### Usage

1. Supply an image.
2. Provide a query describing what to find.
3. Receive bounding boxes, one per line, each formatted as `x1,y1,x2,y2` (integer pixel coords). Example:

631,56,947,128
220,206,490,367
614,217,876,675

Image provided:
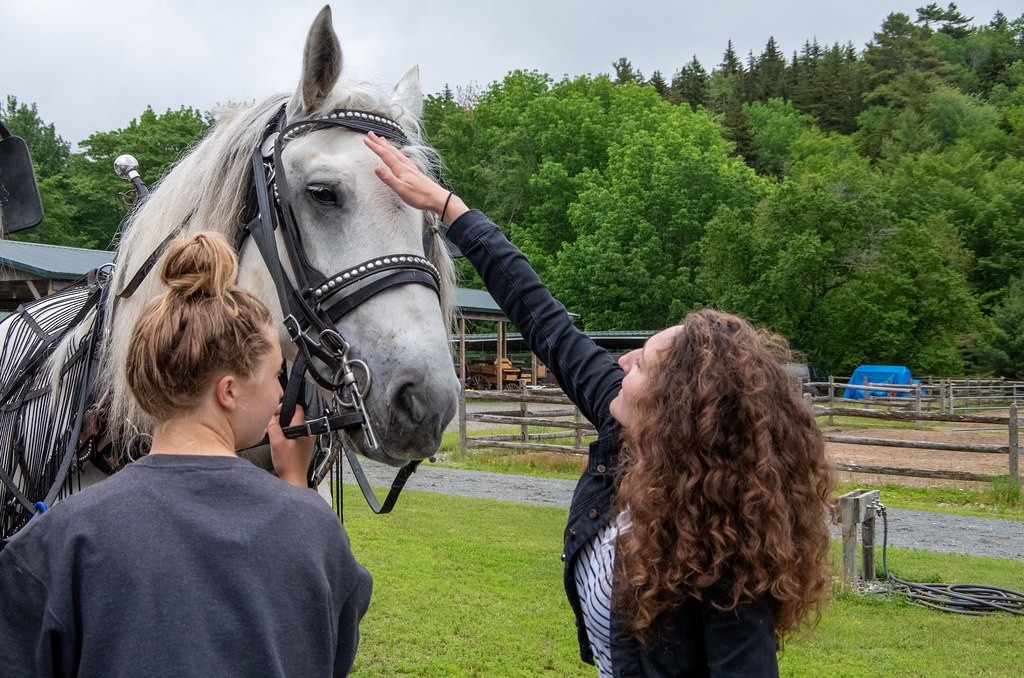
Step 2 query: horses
0,3,463,546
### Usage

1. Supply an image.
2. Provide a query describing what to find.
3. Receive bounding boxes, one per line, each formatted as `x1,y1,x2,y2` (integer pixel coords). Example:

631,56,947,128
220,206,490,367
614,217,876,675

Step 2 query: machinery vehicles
465,357,523,391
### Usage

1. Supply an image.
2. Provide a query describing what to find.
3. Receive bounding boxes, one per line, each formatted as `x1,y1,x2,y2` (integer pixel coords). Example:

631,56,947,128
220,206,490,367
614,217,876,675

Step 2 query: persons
0,230,376,678
360,125,845,678
